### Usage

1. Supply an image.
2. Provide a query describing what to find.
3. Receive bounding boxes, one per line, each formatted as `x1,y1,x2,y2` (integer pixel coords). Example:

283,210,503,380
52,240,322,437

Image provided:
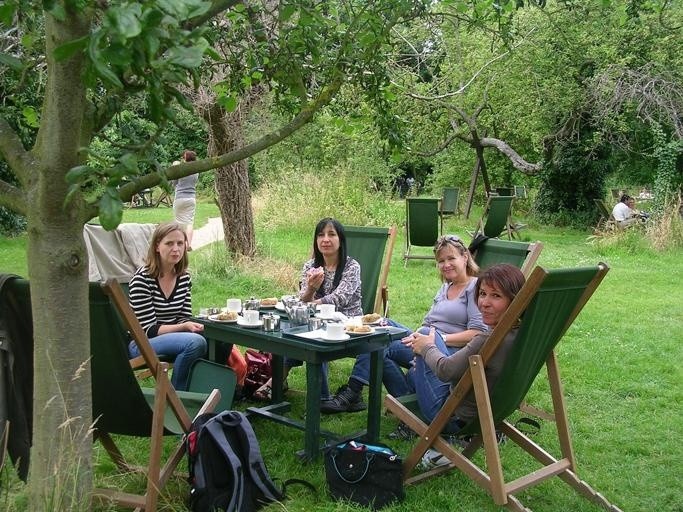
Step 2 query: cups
241,295,335,335
226,299,241,311
326,322,345,338
206,308,220,314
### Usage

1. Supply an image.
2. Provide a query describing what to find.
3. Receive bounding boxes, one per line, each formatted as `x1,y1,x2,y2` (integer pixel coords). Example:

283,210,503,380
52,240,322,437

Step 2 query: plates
208,314,240,323
352,314,383,325
343,325,375,335
219,308,241,313
319,333,350,342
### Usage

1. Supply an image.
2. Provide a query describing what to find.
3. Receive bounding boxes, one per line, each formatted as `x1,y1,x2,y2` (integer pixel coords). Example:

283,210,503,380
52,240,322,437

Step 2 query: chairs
288,224,398,398
0,273,222,511
463,184,530,241
404,196,444,268
438,187,461,220
84,222,159,382
383,261,626,512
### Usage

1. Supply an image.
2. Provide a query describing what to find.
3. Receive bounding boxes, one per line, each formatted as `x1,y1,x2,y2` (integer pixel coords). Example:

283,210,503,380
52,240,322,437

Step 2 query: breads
364,312,382,323
216,310,238,321
354,326,370,333
261,297,278,305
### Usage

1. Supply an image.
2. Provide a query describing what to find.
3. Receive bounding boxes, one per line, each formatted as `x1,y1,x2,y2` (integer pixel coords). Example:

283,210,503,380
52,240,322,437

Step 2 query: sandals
253,378,289,401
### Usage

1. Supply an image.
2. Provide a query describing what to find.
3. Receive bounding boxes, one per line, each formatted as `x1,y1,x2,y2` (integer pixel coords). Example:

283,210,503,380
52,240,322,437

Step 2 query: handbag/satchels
323,438,405,512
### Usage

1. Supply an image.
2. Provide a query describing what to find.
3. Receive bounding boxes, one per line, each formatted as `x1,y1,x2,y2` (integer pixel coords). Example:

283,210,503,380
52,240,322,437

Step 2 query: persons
252,217,363,415
628,196,636,209
410,264,529,468
128,222,209,393
323,236,486,418
406,176,416,192
172,150,199,251
610,194,637,228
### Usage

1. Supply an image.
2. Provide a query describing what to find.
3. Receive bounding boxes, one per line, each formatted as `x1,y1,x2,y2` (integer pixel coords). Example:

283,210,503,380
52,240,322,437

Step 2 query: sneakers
384,421,418,443
412,448,453,473
319,384,366,415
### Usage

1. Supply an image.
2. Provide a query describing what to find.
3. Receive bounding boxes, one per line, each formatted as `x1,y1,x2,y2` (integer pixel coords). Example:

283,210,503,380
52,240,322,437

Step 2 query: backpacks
185,410,286,511
